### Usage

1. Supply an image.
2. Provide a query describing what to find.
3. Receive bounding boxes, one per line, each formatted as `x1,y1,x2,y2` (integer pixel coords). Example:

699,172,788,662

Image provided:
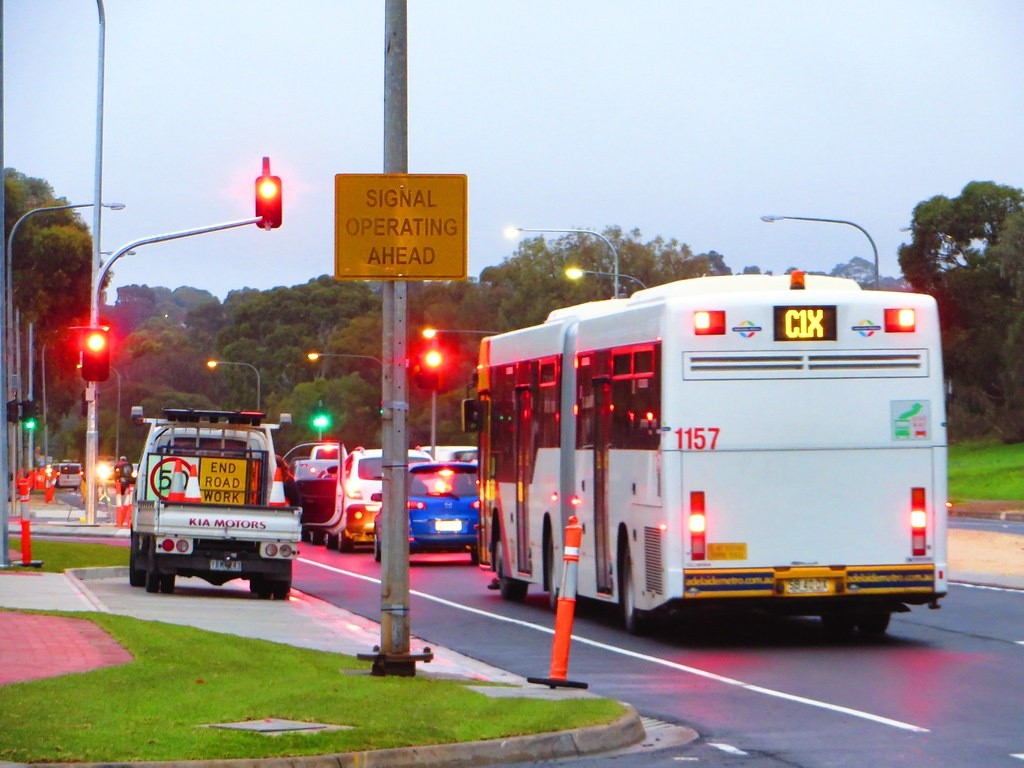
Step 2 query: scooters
112,466,134,494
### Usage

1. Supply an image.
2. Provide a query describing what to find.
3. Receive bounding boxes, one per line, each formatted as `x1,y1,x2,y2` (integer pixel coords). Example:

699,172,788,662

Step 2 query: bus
462,268,951,636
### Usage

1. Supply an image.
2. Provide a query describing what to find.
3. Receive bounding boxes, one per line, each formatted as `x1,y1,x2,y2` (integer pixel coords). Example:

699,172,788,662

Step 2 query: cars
278,443,479,566
36,453,139,494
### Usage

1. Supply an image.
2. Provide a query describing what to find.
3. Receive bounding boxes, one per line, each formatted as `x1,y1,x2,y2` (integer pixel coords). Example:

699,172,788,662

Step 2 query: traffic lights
23,418,38,432
79,326,111,384
255,176,282,230
310,411,333,433
422,348,444,372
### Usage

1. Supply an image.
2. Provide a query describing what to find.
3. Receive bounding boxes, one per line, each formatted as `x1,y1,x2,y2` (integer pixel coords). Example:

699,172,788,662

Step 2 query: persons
114,455,133,494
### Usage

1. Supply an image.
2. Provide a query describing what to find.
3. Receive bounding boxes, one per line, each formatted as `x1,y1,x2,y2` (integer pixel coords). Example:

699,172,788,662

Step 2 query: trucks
127,406,306,602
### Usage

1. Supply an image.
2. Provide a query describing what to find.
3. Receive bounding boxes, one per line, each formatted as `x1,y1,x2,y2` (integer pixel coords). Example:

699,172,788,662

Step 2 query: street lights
514,223,625,299
206,353,264,414
38,323,117,466
6,199,126,480
760,210,883,289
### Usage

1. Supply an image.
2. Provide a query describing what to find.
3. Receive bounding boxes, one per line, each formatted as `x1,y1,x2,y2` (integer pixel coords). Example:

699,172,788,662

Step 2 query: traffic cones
168,459,202,506
269,466,287,507
